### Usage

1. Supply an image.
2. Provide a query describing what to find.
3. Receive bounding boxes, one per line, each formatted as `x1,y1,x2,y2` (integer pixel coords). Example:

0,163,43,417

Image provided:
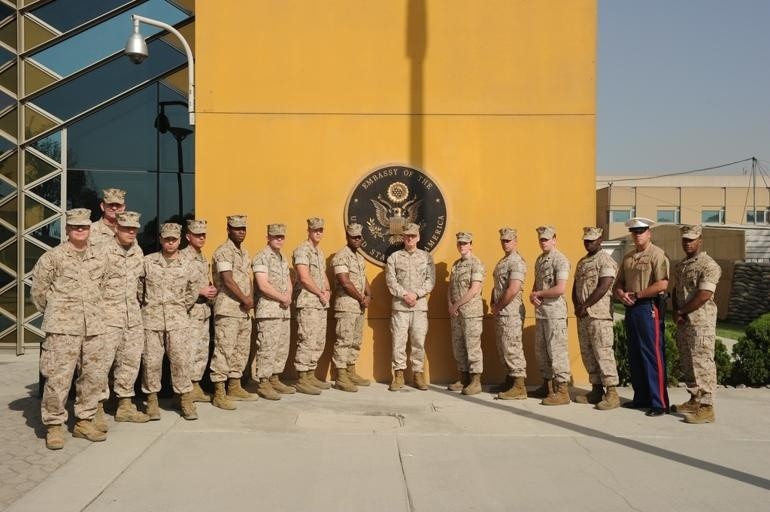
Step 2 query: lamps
125,13,195,126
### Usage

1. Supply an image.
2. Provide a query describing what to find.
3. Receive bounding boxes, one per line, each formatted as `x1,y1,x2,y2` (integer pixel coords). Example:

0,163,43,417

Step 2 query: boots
448,374,623,409
46,388,160,449
413,371,427,389
389,370,405,390
178,365,370,419
670,395,715,424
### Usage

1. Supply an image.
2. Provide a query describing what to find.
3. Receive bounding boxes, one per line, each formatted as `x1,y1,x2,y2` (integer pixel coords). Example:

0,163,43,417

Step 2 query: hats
456,232,472,243
159,224,182,239
269,224,286,236
307,218,324,230
65,207,94,226
402,224,420,235
499,228,516,241
187,220,207,235
536,226,555,240
227,216,247,228
347,224,363,237
103,189,125,204
582,227,603,241
680,225,703,239
626,218,654,232
115,212,141,228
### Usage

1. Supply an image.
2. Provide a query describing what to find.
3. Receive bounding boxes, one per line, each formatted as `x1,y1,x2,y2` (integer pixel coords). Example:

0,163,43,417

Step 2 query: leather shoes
645,407,667,416
622,400,644,409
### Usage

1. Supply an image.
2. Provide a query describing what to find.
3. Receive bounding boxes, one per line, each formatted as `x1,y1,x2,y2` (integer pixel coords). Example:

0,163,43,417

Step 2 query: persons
572,225,622,410
88,211,150,434
251,223,295,400
447,231,485,395
528,224,572,405
330,223,372,394
90,188,138,247
138,221,201,421
671,224,723,425
614,216,672,417
292,217,333,395
210,214,259,410
178,219,217,401
384,222,437,392
490,226,529,400
31,207,107,449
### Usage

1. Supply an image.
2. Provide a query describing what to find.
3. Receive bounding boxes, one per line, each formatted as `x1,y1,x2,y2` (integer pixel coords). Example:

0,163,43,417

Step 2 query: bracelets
632,292,640,302
678,306,681,314
